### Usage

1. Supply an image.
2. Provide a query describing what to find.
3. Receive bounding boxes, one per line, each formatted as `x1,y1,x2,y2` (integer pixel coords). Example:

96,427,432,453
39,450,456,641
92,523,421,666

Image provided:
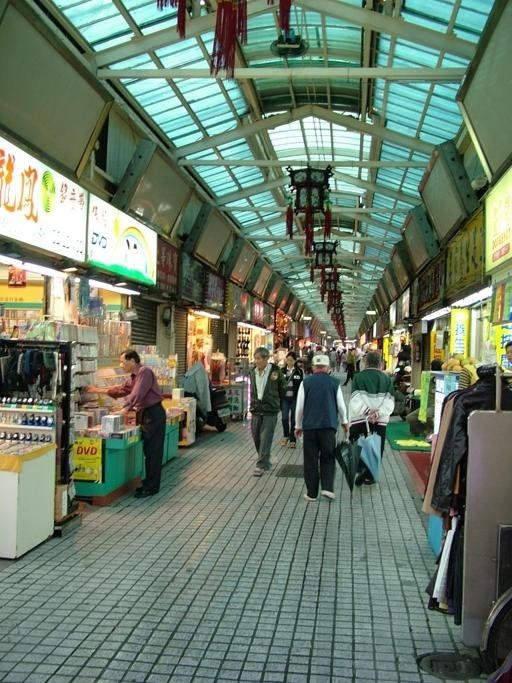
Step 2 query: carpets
383,422,432,500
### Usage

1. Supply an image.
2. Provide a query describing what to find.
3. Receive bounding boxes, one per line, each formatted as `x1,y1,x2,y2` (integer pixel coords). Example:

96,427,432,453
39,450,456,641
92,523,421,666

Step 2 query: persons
86,348,167,497
244,345,395,502
504,340,512,367
393,359,405,375
403,359,411,375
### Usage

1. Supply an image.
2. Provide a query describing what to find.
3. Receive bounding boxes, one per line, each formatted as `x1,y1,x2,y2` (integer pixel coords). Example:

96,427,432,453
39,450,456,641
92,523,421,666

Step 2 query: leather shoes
135,486,160,499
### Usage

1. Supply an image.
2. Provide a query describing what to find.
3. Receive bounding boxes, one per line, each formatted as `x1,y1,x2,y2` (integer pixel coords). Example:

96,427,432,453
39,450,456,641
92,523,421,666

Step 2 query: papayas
441,354,478,372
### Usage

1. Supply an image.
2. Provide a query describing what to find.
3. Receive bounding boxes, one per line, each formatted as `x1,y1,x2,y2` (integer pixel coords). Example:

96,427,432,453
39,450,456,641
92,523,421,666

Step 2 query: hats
311,354,331,368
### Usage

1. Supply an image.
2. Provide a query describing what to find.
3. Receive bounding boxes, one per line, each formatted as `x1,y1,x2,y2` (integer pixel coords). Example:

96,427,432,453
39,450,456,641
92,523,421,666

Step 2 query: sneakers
303,494,317,501
320,489,336,500
280,436,296,449
254,466,269,477
354,468,375,485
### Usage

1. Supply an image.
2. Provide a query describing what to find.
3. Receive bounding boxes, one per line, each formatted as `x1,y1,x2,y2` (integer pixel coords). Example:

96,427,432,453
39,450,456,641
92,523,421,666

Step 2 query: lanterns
327,293,349,347
286,164,333,221
310,240,337,269
319,272,340,292
